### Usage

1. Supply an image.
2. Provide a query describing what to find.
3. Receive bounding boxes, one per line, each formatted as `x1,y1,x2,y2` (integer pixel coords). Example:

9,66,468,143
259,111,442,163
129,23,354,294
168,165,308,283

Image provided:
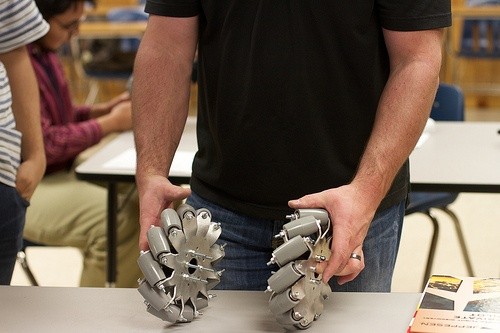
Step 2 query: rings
350,253,361,260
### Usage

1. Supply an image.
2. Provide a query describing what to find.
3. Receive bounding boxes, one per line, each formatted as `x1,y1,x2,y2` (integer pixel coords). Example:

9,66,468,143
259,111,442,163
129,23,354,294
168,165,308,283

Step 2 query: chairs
402,83,476,292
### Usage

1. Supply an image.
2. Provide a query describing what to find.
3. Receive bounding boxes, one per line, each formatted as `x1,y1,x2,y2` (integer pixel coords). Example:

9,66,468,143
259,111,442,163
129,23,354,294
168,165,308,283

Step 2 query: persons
129,1,453,289
22,5,141,288
1,1,50,287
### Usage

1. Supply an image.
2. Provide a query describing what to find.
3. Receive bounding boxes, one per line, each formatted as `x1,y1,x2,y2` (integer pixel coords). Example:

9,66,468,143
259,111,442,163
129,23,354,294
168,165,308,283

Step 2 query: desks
75,116,500,294
0,285,423,333
